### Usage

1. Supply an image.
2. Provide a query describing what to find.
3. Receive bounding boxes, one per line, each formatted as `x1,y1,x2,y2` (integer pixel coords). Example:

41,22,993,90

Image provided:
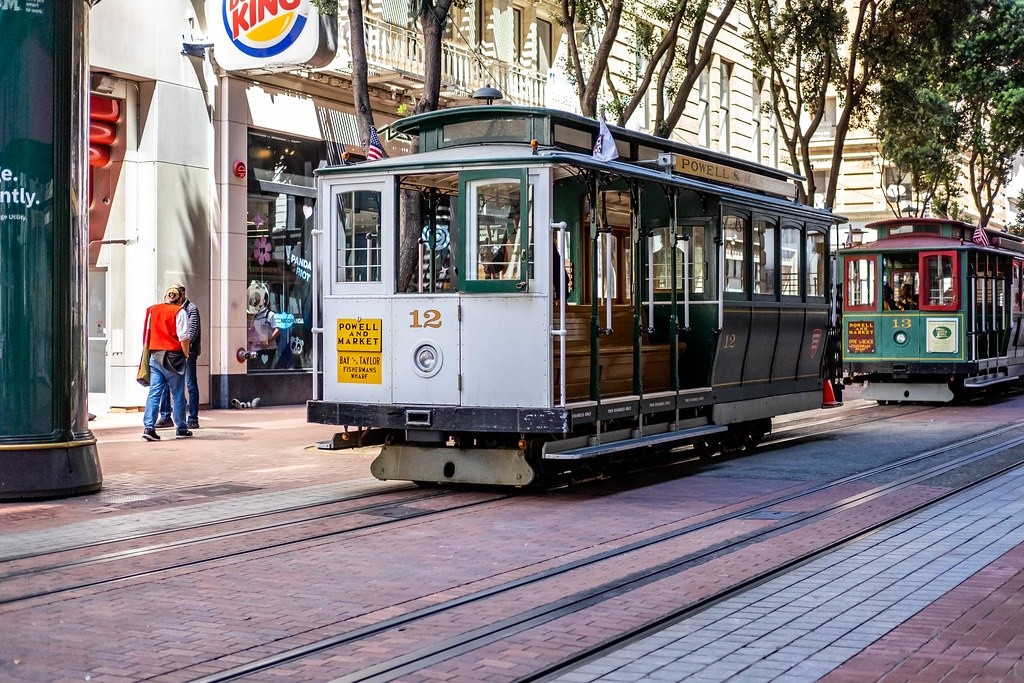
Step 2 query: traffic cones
823,378,838,407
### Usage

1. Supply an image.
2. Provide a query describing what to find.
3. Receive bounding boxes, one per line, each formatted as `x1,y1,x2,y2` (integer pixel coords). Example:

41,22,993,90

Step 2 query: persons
883,283,899,310
248,289,280,368
898,284,917,310
142,287,193,442
153,283,201,429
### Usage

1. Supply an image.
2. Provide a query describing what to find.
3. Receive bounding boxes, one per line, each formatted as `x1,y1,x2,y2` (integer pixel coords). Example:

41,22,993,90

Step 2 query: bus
839,217,1024,405
250,230,313,368
304,85,847,495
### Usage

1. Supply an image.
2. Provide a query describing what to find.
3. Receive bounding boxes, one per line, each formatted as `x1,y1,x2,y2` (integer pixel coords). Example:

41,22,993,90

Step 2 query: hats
163,287,181,303
245,283,270,314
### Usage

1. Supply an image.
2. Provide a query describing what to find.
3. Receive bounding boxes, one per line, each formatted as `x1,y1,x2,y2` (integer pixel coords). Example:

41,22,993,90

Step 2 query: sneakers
186,421,199,429
175,429,194,438
153,418,174,428
141,428,161,441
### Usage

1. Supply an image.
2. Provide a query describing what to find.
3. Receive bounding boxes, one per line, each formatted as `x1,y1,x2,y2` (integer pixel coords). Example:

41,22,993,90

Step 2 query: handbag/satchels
136,345,151,387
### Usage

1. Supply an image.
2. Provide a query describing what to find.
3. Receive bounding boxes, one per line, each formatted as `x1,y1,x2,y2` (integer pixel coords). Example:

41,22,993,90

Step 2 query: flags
591,114,619,162
367,127,383,161
973,220,989,247
846,226,853,245
